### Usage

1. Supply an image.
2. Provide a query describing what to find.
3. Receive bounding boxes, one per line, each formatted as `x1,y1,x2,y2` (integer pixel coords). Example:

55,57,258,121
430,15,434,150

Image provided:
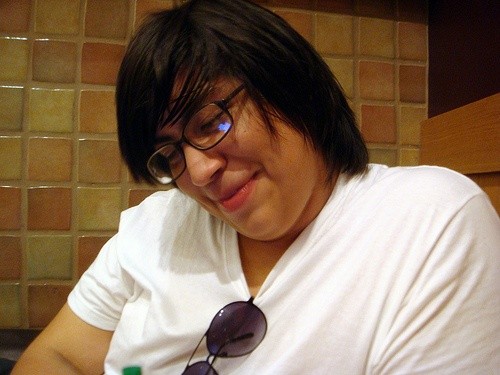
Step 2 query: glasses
147,82,249,187
181,296,267,375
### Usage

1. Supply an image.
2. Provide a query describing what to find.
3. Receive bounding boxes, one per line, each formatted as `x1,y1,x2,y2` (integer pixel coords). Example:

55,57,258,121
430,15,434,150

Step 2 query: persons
7,1,500,375
